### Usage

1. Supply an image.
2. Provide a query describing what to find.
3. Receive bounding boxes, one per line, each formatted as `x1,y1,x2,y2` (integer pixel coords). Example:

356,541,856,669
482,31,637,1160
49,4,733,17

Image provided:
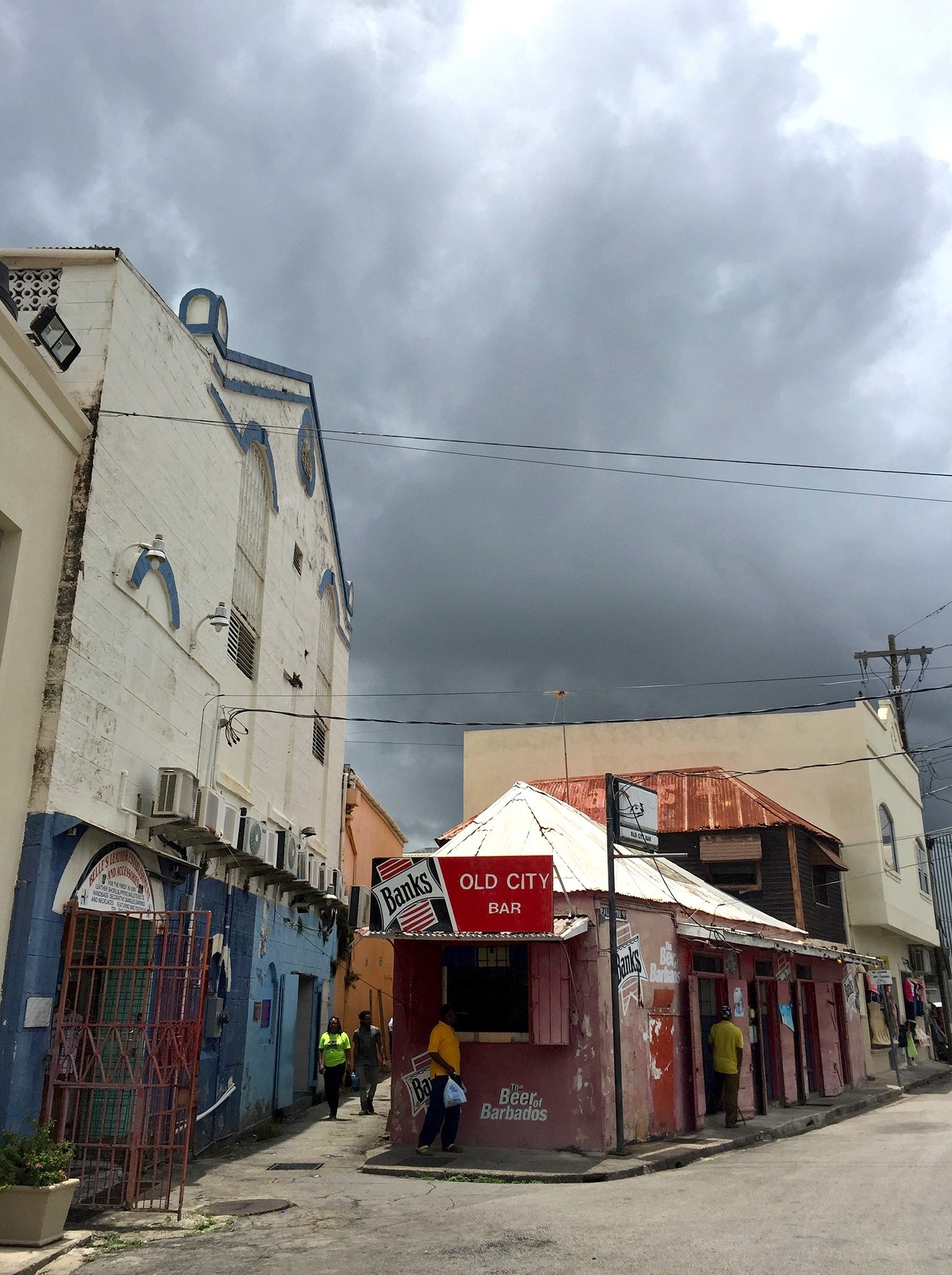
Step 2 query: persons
318,1016,352,1121
905,1021,919,1068
353,1010,387,1115
708,1005,744,1130
416,1005,464,1156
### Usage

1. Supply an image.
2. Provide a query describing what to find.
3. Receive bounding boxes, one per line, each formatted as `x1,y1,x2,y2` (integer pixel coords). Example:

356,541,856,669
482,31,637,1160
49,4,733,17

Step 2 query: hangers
907,1027,911,1033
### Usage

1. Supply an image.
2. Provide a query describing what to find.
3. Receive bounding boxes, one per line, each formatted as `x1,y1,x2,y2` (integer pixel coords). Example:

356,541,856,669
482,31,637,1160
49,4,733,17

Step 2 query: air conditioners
153,767,346,901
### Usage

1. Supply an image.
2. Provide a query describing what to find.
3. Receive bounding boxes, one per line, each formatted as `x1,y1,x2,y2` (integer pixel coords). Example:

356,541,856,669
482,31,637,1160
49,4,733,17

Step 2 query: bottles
352,1072,358,1087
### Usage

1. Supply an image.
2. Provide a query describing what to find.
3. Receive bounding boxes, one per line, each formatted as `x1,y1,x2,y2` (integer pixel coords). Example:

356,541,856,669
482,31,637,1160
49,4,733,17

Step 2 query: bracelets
383,1057,385,1060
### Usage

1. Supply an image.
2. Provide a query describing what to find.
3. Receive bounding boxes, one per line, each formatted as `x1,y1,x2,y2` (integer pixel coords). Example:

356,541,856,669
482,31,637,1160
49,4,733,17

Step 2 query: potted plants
0,1115,80,1248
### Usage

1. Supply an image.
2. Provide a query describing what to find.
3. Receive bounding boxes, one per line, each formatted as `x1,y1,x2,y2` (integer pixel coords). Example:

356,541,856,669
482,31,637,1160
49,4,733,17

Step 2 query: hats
720,1006,732,1018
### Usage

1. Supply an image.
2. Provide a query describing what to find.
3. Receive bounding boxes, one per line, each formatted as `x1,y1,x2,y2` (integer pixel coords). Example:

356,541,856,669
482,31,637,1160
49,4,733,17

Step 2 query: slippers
415,1145,433,1155
442,1144,462,1153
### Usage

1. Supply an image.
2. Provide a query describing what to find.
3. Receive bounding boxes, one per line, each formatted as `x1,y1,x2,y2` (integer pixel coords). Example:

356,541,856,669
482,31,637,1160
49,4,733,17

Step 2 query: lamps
303,882,337,905
114,533,166,576
191,601,228,649
22,306,82,374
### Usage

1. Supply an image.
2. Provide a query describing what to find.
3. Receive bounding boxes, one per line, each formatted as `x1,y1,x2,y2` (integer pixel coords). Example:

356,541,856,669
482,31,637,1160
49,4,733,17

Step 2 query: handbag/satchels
351,1072,358,1088
443,1076,468,1108
344,1074,353,1088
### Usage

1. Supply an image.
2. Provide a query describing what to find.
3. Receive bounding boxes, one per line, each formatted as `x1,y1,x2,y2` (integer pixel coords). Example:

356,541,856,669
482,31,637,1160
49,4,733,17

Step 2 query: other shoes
367,1102,374,1113
326,1113,336,1121
725,1122,739,1128
359,1107,368,1115
